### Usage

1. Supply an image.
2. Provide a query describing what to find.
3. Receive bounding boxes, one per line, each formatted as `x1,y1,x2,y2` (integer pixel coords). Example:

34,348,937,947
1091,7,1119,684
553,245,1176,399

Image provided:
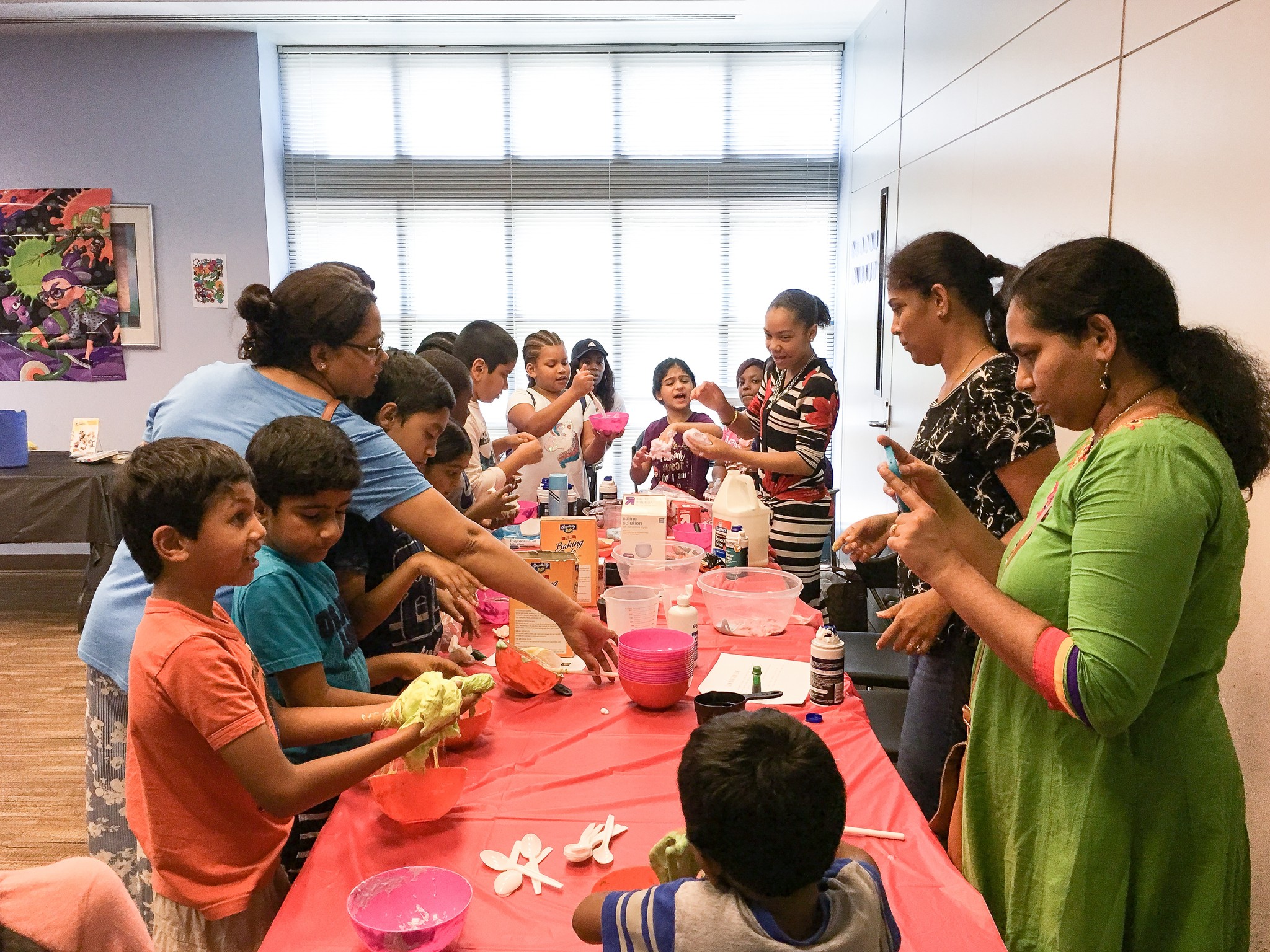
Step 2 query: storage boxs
509,550,579,658
668,485,703,528
540,516,598,607
620,494,668,562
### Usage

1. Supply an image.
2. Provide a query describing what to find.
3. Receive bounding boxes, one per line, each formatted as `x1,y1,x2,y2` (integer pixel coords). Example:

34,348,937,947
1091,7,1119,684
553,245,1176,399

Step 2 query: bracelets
719,406,738,426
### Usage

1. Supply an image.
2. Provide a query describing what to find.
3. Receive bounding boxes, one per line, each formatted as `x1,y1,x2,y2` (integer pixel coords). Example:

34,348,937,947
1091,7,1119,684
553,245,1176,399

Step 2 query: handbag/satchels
928,737,967,873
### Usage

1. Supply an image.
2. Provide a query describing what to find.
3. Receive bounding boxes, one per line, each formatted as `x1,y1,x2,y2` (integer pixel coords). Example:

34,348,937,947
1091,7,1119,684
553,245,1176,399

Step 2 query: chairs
837,627,912,760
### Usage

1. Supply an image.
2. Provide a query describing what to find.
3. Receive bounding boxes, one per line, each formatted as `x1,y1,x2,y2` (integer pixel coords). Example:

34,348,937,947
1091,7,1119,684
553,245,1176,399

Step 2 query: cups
603,505,622,537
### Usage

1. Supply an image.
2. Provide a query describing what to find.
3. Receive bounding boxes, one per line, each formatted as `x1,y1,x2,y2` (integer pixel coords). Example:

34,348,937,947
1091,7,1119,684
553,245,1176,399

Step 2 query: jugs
600,585,670,638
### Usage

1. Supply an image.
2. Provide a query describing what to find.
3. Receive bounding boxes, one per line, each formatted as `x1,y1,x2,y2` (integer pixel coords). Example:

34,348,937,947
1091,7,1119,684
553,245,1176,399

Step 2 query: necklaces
767,349,817,410
1087,384,1167,451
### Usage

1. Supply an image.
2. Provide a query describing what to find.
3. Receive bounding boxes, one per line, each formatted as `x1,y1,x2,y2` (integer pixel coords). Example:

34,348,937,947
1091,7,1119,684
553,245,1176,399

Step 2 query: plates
591,866,660,894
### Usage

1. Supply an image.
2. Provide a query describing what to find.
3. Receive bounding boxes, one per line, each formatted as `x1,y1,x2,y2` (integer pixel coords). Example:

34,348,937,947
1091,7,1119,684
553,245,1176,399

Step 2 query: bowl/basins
346,866,473,952
589,412,629,436
671,523,712,549
704,489,760,502
697,567,804,638
611,540,706,601
496,638,565,696
513,501,538,524
474,588,510,625
368,767,467,824
618,628,695,709
439,695,493,751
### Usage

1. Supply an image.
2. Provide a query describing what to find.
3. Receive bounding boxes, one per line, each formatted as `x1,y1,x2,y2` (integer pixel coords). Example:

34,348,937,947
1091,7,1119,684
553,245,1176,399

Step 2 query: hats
571,338,608,361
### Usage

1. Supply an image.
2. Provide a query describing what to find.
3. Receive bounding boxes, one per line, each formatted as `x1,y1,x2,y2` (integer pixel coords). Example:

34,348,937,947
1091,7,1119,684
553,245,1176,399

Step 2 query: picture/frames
111,204,161,349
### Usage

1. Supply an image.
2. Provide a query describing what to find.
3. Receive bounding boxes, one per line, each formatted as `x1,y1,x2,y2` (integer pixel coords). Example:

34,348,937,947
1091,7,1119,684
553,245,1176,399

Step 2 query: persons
686,289,840,629
414,319,544,554
231,416,468,882
572,707,901,952
118,439,482,951
77,266,619,938
324,347,481,695
878,237,1270,952
565,339,627,468
505,330,624,502
704,358,764,503
832,232,1061,850
630,358,723,501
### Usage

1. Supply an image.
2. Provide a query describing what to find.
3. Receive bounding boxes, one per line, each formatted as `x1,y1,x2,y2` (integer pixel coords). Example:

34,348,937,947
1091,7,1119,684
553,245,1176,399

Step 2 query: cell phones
885,445,909,513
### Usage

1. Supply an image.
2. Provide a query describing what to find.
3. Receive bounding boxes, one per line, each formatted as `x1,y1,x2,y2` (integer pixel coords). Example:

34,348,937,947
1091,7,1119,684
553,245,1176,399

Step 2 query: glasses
333,331,386,356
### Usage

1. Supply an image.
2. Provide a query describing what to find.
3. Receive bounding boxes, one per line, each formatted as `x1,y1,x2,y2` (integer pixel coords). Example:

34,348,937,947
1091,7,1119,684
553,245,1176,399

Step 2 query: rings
891,523,899,534
910,641,920,649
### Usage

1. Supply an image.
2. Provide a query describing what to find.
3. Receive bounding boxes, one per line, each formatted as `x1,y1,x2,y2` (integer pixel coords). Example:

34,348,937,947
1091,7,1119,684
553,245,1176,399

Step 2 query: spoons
520,833,542,894
479,849,563,888
494,847,553,894
563,814,628,864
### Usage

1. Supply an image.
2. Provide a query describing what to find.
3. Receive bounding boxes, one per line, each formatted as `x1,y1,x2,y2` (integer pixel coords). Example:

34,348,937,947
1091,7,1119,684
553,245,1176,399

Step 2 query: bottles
668,584,698,668
599,476,617,501
725,525,749,581
752,666,761,693
711,467,770,567
809,625,844,707
536,473,577,518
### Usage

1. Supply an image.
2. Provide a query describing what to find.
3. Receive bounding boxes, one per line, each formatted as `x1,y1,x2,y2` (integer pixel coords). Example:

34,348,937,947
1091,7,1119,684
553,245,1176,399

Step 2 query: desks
258,560,1005,952
0,451,122,634
